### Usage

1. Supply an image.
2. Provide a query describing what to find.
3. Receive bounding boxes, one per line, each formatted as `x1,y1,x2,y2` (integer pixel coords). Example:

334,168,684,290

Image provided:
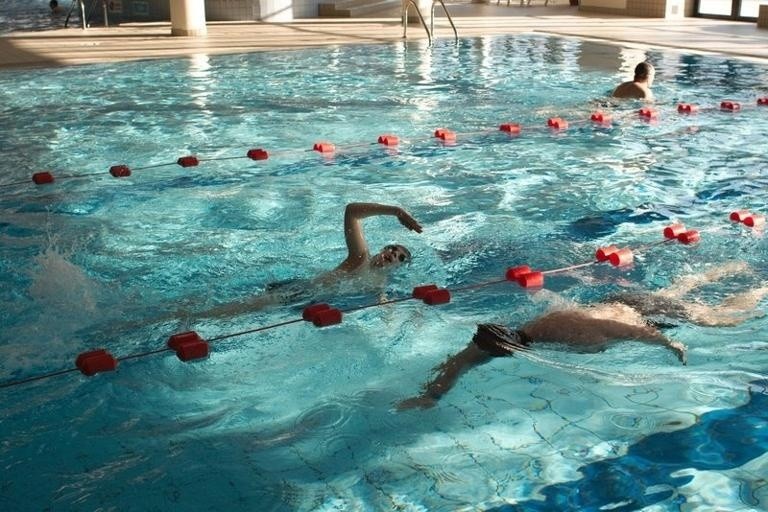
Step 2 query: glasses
385,245,408,263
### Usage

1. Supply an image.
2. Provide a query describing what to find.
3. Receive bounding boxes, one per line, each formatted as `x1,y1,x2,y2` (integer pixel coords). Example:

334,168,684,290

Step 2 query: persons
395,260,767,411
613,62,655,101
105,202,422,335
